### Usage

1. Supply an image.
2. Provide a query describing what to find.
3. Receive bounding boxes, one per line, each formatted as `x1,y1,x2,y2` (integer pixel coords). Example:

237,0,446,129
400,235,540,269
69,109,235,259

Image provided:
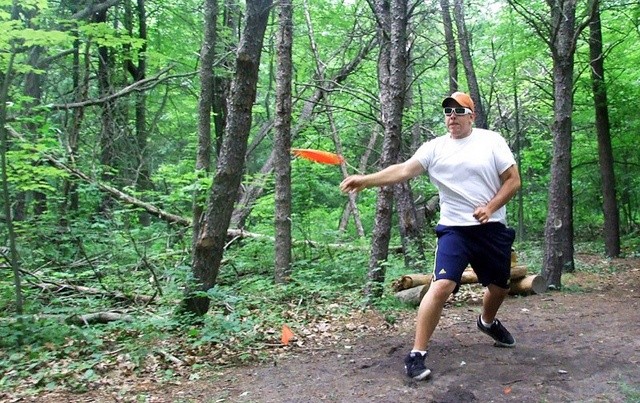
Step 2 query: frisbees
289,147,343,165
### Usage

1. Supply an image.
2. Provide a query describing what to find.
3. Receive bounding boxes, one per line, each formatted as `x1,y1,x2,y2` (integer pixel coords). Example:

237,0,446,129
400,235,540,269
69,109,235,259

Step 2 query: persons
339,91,522,382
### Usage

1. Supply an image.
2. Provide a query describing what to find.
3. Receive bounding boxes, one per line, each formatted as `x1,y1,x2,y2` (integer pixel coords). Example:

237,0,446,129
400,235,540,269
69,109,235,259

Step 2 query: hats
442,92,474,112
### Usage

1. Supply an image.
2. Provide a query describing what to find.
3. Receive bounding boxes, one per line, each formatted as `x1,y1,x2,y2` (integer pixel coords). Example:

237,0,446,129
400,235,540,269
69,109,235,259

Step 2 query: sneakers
404,350,431,381
476,314,516,347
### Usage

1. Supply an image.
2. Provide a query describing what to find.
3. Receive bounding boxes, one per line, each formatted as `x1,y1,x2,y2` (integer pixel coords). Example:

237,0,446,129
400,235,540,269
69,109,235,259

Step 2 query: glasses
442,106,472,116
